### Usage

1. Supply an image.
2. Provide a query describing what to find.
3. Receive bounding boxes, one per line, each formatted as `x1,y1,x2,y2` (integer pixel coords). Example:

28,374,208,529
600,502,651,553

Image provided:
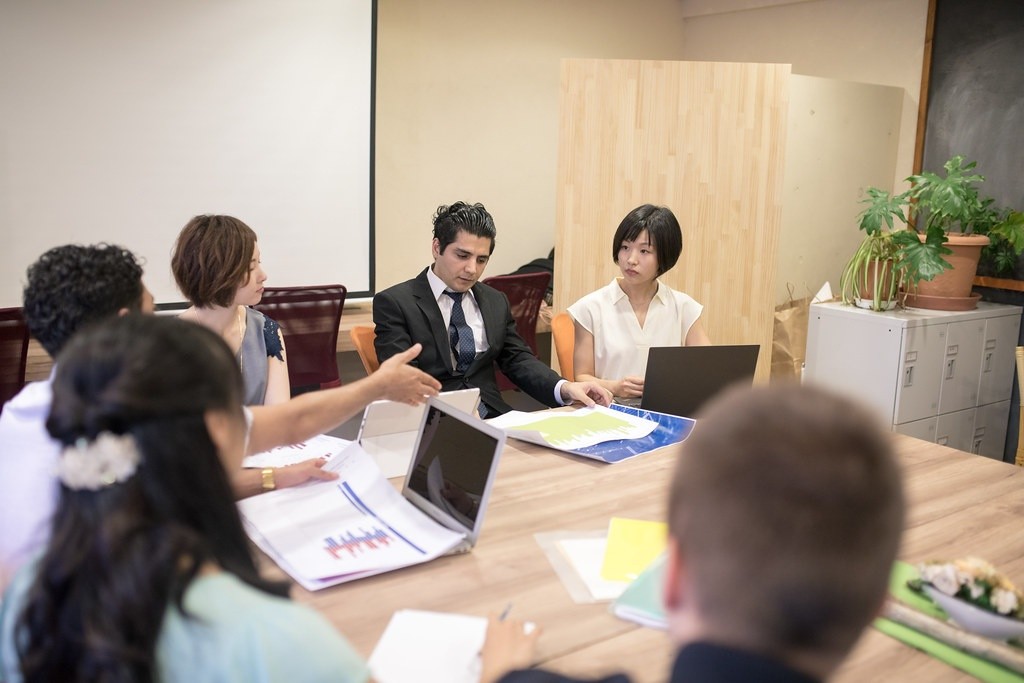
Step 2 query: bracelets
259,467,275,492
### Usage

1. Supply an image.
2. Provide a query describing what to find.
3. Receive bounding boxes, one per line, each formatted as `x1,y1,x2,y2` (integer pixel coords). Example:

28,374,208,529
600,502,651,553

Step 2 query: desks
280,297,551,375
249,406,1024,683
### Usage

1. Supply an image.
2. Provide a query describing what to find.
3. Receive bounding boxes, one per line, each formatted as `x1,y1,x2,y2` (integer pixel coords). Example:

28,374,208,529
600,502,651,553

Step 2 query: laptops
401,395,506,557
611,345,761,421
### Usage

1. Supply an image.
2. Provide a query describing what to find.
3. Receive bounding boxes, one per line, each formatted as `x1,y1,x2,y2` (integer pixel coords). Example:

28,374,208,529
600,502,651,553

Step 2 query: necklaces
193,303,243,375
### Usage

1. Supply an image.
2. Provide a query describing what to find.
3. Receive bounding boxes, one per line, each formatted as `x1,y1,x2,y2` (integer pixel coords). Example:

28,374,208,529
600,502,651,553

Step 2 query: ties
442,290,488,421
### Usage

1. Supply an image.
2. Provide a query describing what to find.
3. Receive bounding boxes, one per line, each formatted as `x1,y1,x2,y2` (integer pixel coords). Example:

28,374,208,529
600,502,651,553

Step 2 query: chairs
247,283,345,396
482,271,552,391
0,307,31,416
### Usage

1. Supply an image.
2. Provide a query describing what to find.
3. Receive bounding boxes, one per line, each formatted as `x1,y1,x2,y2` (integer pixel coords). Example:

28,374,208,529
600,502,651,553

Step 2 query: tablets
358,388,480,446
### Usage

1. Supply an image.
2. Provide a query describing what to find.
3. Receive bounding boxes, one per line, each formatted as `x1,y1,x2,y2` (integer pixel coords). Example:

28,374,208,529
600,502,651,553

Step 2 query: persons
163,214,292,407
373,201,614,420
0,240,442,561
1,311,381,683
488,369,906,683
567,203,709,400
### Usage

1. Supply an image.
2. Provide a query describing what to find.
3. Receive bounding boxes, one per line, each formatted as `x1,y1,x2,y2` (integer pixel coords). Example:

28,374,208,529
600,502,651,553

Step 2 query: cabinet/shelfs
806,296,1023,462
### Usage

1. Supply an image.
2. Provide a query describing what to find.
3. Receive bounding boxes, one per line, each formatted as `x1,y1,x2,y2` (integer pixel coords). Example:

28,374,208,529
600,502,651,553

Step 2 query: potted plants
852,150,1013,311
840,231,902,309
